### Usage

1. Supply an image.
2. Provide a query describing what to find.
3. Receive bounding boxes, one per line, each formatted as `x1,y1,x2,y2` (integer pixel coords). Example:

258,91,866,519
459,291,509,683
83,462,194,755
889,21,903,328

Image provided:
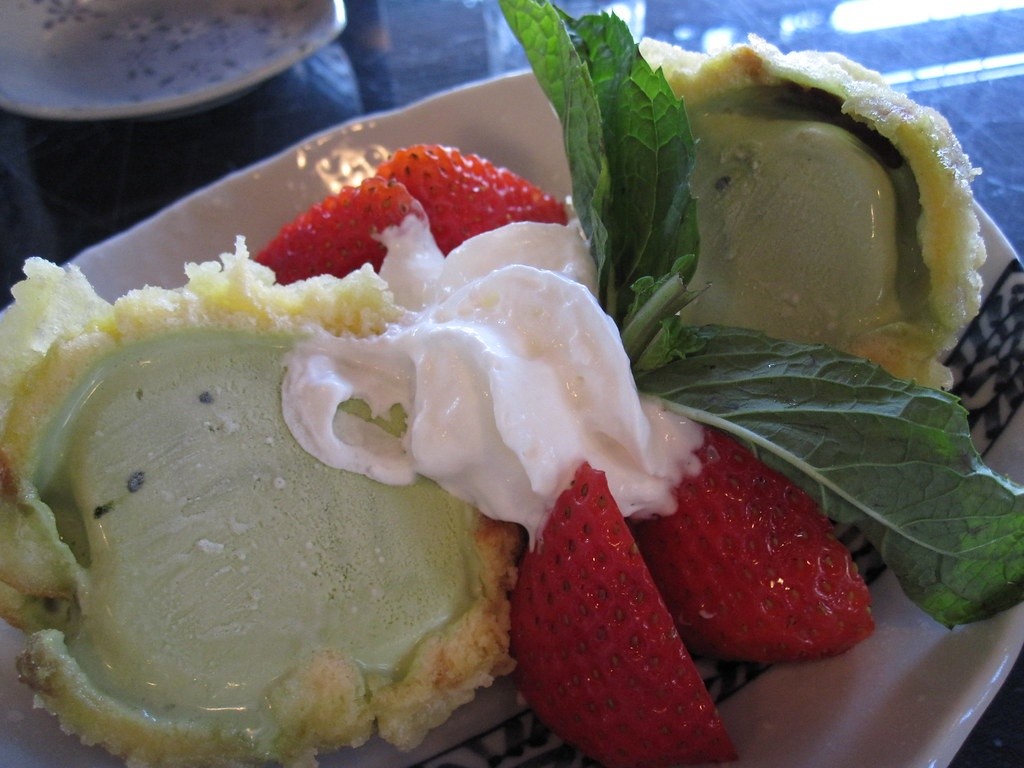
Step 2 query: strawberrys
248,175,431,286
506,459,735,768
637,398,877,670
368,145,573,256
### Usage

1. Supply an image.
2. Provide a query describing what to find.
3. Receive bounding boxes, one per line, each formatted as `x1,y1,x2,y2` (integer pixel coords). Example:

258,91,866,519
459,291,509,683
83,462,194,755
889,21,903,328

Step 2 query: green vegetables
506,0,1023,646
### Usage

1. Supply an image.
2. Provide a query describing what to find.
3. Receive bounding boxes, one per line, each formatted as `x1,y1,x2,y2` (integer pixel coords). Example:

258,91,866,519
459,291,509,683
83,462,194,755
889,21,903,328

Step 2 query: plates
1,0,349,125
0,67,1024,767
